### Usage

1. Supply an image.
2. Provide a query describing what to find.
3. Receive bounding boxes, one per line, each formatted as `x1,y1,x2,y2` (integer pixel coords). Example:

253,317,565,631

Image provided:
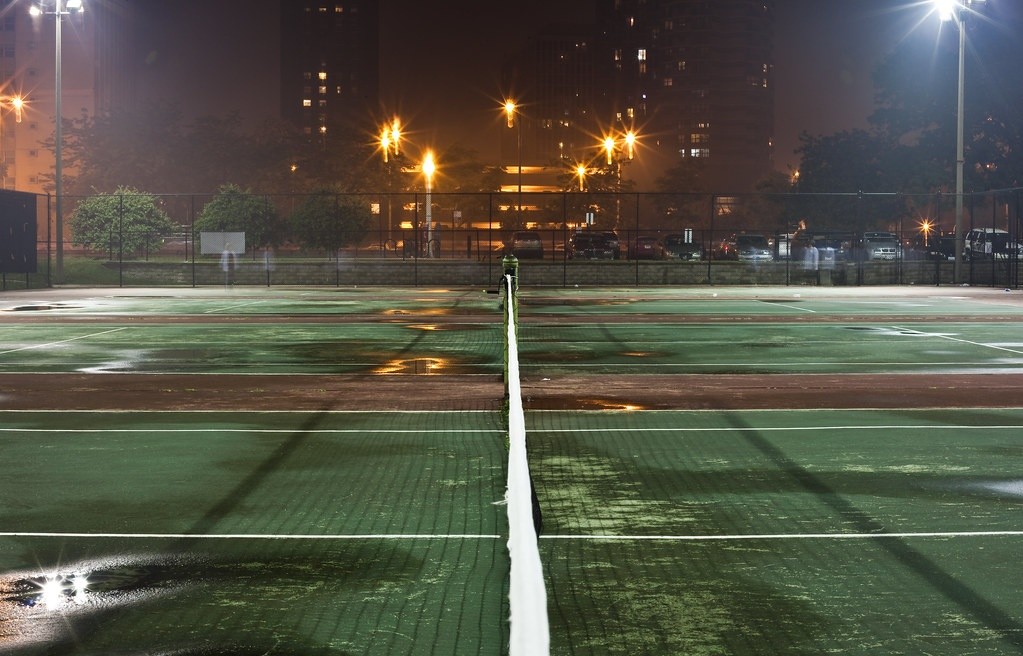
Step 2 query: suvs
507,230,548,261
902,227,1023,261
564,232,622,262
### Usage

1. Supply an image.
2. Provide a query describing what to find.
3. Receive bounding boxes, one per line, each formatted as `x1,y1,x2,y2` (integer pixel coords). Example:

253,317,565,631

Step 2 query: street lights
28,0,85,284
934,0,969,285
381,126,401,251
422,149,436,259
503,99,523,229
605,131,634,230
923,222,929,247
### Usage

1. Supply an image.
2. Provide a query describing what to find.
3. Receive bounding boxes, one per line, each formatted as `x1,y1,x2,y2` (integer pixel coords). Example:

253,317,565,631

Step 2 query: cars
626,234,705,264
767,232,905,264
714,234,773,263
